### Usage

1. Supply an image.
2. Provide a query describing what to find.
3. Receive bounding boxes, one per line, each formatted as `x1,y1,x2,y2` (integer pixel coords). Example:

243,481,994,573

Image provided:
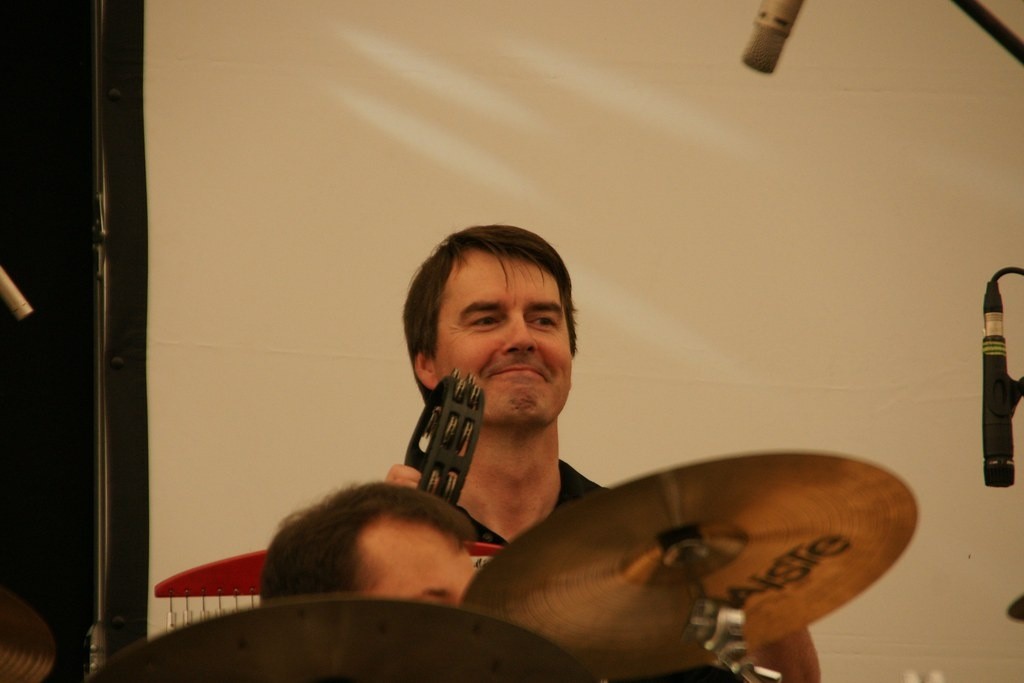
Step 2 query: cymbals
81,592,596,683
0,587,56,683
403,368,485,506
458,449,920,680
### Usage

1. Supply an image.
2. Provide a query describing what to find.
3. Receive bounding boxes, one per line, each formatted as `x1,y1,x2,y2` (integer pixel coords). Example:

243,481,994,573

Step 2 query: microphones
981,281,1016,491
743,1,804,74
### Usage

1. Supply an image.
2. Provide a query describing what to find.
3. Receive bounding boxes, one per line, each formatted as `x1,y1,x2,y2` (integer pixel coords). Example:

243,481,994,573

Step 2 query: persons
382,226,824,683
257,479,478,608
